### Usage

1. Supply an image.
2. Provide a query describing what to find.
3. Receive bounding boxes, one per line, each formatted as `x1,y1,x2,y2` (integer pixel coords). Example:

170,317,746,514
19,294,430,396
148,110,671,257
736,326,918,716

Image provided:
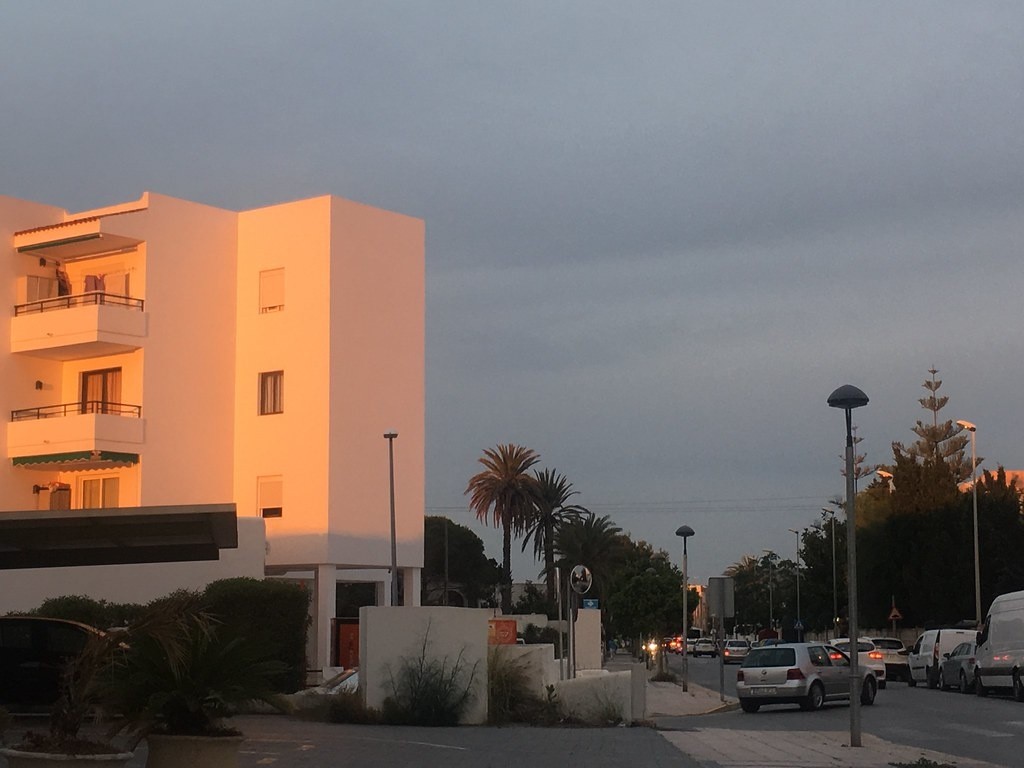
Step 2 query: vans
975,590,1024,700
906,629,977,687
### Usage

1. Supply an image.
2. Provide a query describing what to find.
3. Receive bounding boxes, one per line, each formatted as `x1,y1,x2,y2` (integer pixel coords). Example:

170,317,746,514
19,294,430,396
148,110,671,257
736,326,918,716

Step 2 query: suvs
826,638,888,690
736,642,878,714
862,636,909,681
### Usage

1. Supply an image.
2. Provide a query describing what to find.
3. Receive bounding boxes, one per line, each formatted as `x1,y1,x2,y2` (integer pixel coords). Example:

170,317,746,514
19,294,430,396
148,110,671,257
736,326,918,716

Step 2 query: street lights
382,429,400,605
675,525,696,693
823,507,839,639
825,384,869,749
788,528,803,644
954,418,982,632
763,549,773,631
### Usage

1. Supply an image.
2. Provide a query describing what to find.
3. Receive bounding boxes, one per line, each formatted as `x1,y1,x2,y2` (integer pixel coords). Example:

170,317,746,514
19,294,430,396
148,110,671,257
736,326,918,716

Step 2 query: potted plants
0,586,250,768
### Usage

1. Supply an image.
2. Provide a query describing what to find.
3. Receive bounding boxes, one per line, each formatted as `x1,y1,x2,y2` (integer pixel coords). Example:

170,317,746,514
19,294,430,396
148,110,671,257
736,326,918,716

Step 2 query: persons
614,636,619,655
609,637,615,660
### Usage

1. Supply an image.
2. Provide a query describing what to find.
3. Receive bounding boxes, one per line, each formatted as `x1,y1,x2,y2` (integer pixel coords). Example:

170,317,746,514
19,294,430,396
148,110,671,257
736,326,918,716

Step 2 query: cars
642,633,790,663
294,666,360,704
0,614,137,713
937,641,977,693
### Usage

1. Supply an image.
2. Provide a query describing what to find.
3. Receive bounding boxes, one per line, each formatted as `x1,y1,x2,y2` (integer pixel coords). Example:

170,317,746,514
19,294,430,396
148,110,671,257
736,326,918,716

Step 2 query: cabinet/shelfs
38,487,70,511
17,275,59,315
